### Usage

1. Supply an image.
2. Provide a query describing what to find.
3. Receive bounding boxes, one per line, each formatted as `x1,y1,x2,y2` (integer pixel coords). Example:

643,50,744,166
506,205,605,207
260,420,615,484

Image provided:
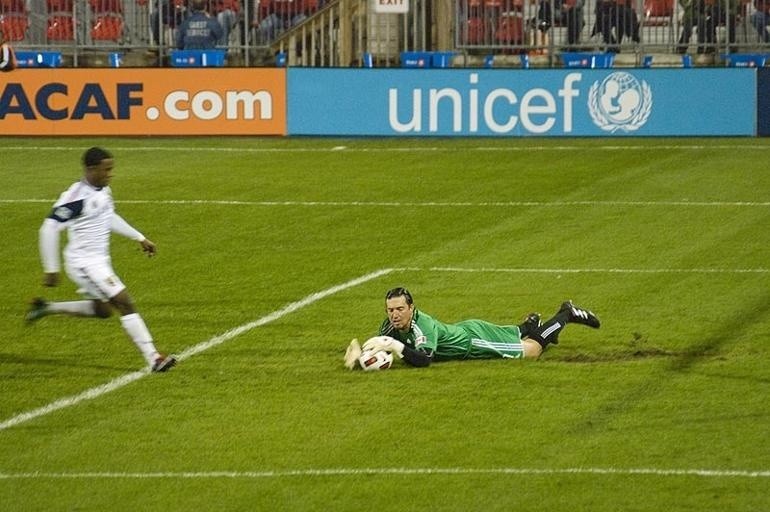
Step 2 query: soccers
359,343,393,371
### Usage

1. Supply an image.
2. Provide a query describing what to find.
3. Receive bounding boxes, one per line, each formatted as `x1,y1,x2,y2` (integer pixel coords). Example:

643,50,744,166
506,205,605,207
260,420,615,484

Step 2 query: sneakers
152,355,176,372
23,295,49,321
525,312,544,332
560,300,601,328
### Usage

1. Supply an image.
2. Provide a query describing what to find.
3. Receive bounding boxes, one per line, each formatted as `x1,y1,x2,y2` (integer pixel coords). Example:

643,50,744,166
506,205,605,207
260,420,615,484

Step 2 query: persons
1,30,20,73
24,148,177,371
463,1,641,53
343,287,601,372
152,1,322,50
643,1,768,54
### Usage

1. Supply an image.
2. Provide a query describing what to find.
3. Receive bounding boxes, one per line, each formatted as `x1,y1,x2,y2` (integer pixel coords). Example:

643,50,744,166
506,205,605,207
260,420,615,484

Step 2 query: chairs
460,0,677,52
0,1,322,43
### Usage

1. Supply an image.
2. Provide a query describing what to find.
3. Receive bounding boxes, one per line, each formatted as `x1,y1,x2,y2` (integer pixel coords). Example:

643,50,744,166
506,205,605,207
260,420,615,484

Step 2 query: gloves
361,335,405,359
343,338,362,370
